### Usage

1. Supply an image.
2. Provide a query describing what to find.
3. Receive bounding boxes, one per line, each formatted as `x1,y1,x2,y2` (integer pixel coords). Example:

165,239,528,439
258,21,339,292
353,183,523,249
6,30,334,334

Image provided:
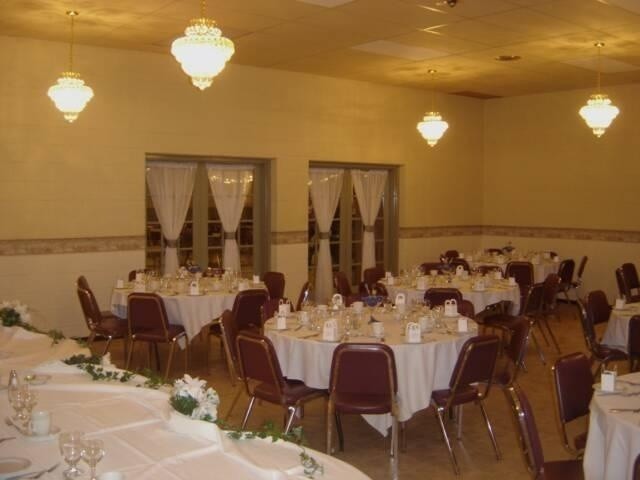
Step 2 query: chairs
218,246,638,476
77,266,286,382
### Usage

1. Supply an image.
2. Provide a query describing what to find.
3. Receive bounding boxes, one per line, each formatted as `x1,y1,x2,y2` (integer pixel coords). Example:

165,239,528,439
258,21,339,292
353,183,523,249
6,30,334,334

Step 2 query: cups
29,411,52,435
394,265,516,289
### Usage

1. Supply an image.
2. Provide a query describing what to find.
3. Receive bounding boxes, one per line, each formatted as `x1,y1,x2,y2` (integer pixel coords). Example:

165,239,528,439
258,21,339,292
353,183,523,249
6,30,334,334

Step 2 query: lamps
414,67,452,150
46,10,96,126
575,42,623,142
170,0,238,94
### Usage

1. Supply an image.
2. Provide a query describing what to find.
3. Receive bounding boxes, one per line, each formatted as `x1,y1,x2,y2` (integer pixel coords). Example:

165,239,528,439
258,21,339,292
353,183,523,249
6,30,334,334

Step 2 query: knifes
299,333,319,339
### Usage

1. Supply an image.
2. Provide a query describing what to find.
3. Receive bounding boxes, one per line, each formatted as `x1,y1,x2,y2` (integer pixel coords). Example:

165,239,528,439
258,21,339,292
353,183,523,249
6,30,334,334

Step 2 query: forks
615,380,640,386
6,418,27,435
598,391,633,398
5,462,60,480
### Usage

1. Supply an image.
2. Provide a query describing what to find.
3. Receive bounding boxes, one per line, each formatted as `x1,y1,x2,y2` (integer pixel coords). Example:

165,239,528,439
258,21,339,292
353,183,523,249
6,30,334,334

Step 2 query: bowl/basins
23,373,53,386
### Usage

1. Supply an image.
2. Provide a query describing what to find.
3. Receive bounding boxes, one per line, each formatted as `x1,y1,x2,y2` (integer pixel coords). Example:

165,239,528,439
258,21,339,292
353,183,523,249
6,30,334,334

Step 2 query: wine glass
79,439,107,480
143,265,241,295
303,294,445,339
57,431,85,480
7,383,38,430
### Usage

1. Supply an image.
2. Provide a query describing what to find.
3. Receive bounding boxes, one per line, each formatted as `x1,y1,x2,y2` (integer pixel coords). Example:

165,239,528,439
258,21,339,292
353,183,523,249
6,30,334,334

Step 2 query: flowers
1,297,30,325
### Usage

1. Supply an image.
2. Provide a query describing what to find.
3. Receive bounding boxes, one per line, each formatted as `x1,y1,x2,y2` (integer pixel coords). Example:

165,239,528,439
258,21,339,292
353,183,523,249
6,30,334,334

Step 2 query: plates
591,383,630,394
0,454,46,480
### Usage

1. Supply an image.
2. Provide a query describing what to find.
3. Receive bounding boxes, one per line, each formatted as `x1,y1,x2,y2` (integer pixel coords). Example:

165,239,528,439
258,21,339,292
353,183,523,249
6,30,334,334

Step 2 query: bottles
7,368,20,393
238,277,249,292
